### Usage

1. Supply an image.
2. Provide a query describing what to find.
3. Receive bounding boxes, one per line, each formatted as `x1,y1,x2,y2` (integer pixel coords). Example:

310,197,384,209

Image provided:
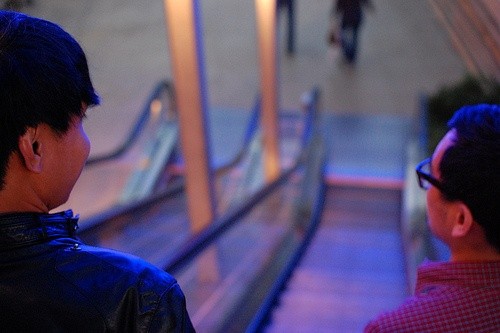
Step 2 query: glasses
417,159,448,196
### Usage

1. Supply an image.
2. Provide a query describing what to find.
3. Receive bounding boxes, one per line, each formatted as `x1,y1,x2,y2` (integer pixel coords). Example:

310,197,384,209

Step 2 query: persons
328,0,371,62
362,104,500,332
1,11,194,333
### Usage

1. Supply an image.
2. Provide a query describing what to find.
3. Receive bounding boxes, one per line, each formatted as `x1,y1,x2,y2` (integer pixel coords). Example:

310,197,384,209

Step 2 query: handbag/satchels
327,32,336,44
